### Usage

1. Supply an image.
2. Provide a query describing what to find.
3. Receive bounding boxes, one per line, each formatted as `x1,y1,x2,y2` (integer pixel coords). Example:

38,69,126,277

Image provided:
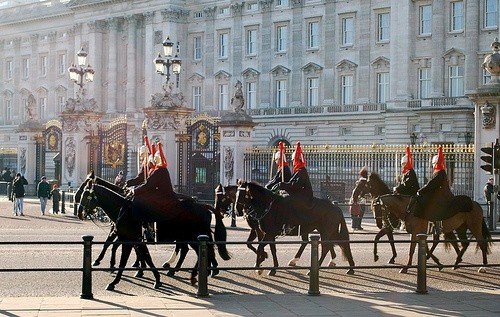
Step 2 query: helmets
432,147,444,173
148,144,159,170
154,142,168,169
275,143,289,168
291,141,306,173
140,136,152,162
401,147,413,173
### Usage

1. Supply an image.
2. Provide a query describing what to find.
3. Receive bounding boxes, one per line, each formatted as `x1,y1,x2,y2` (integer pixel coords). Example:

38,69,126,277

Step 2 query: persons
0,165,16,194
414,154,454,218
14,173,29,215
148,153,155,175
126,151,178,236
348,180,369,230
48,184,61,215
12,173,22,214
271,151,313,234
120,146,155,193
263,151,292,197
393,154,419,195
484,177,494,224
114,170,125,189
37,176,51,215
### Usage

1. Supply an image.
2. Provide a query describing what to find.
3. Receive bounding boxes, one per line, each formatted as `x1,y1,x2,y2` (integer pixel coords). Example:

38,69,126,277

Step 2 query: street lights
153,37,180,95
67,47,95,99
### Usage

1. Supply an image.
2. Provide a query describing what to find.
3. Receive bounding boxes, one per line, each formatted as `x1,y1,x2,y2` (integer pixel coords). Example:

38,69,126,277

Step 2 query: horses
74,170,233,292
213,179,355,275
349,165,497,273
